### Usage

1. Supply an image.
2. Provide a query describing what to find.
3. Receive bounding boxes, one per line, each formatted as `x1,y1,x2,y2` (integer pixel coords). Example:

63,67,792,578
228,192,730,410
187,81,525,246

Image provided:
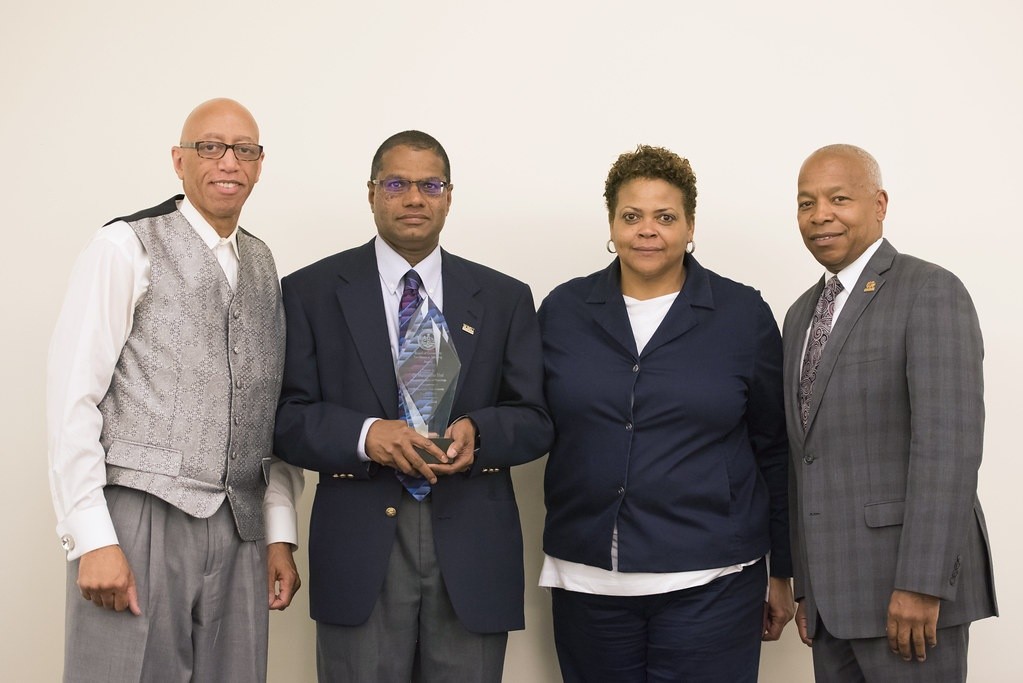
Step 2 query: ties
800,277,844,430
397,269,438,502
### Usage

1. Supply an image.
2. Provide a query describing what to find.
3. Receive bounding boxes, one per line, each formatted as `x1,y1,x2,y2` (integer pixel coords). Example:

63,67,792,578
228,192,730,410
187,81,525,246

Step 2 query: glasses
180,140,263,161
372,177,448,197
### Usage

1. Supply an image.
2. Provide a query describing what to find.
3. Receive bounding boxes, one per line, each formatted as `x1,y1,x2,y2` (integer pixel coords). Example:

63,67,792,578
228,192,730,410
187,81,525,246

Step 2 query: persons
782,143,999,683
274,129,554,683
538,146,796,683
49,98,305,683
